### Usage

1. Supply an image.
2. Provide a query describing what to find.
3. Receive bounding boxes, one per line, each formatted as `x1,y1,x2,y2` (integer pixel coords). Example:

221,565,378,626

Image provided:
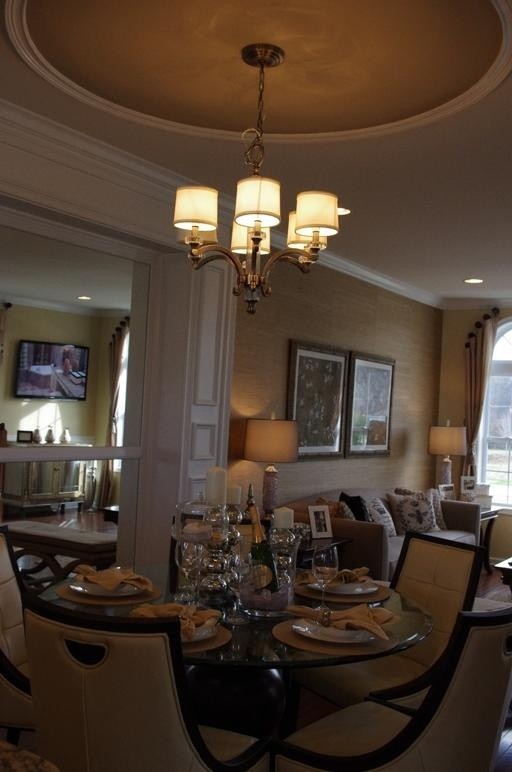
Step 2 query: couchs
279,487,480,581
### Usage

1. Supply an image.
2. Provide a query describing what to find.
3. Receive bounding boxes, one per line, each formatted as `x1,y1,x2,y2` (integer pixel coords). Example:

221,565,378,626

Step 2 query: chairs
0,525,37,743
289,528,484,706
268,608,511,772
22,606,273,772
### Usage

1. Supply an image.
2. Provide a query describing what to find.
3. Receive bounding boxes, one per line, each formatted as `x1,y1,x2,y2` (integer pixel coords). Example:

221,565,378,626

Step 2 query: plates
293,618,382,643
180,626,215,644
68,581,138,595
309,583,378,595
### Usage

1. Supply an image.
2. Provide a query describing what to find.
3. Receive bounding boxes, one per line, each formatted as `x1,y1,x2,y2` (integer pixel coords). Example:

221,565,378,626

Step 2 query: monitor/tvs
13,339,91,401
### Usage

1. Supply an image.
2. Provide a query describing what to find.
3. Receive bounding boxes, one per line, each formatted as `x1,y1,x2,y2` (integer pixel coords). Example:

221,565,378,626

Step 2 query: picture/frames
460,475,477,494
285,339,349,459
438,483,456,501
17,431,33,442
343,350,396,458
307,503,333,540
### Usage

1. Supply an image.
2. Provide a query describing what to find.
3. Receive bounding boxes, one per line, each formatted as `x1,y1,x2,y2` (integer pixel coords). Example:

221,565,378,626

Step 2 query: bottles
248,505,279,595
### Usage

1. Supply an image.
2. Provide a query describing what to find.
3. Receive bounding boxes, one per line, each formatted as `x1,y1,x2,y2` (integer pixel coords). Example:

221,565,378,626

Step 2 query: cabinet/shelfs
1,440,93,509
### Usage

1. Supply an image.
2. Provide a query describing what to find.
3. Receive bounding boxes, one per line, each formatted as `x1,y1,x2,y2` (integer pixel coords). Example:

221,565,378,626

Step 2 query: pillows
395,488,447,530
367,497,396,536
383,492,440,535
316,496,356,520
339,491,374,522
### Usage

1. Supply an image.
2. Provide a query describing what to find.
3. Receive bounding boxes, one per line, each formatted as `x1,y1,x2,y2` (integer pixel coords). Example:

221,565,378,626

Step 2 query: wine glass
312,546,338,613
175,542,204,602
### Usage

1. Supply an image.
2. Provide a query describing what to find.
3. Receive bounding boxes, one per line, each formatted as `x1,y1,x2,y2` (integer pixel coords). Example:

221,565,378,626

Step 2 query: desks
241,515,353,568
0,509,117,601
99,505,118,525
480,509,499,575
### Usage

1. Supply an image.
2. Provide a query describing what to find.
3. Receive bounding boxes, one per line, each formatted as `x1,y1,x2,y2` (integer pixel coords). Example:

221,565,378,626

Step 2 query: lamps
241,417,299,528
173,44,341,316
428,426,467,484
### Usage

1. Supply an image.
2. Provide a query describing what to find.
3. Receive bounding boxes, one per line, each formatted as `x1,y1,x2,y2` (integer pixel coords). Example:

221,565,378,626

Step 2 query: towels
71,563,154,594
128,602,223,640
296,566,372,587
283,601,401,643
